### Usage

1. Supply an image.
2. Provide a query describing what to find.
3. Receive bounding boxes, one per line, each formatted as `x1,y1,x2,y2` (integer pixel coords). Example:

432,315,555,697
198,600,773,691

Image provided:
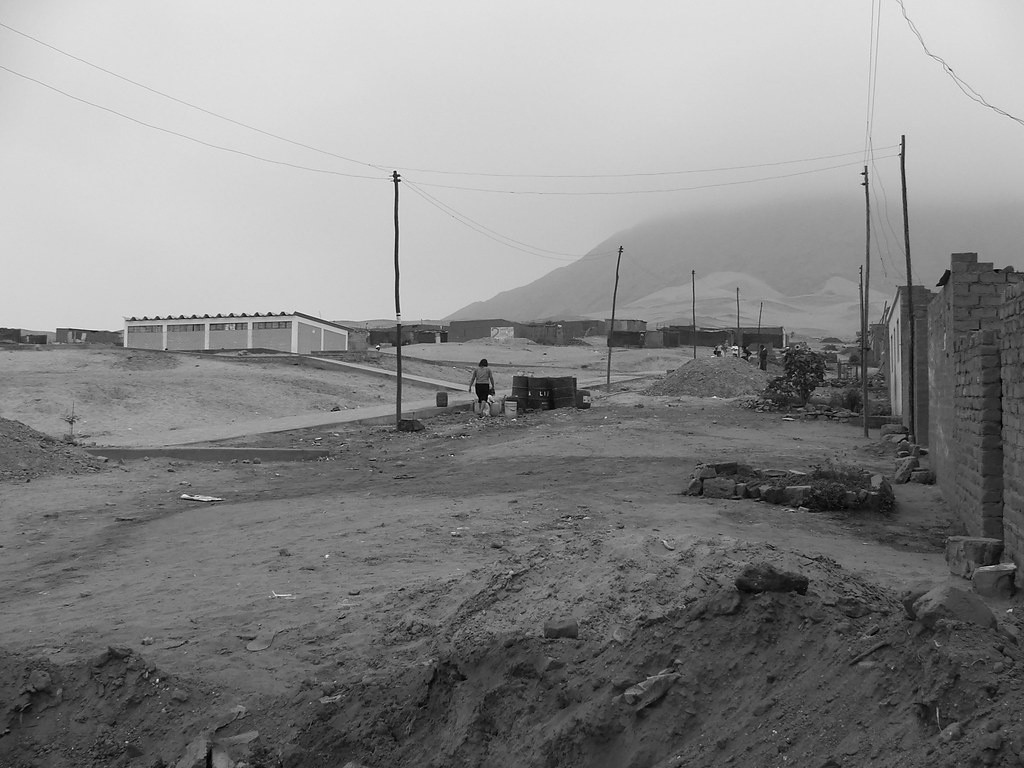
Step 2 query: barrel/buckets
505,402,517,419
512,375,591,408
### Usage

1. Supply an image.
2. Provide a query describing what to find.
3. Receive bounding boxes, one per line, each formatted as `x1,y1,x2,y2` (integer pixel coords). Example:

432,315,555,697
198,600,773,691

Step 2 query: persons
469,359,495,418
758,345,767,370
713,338,752,362
